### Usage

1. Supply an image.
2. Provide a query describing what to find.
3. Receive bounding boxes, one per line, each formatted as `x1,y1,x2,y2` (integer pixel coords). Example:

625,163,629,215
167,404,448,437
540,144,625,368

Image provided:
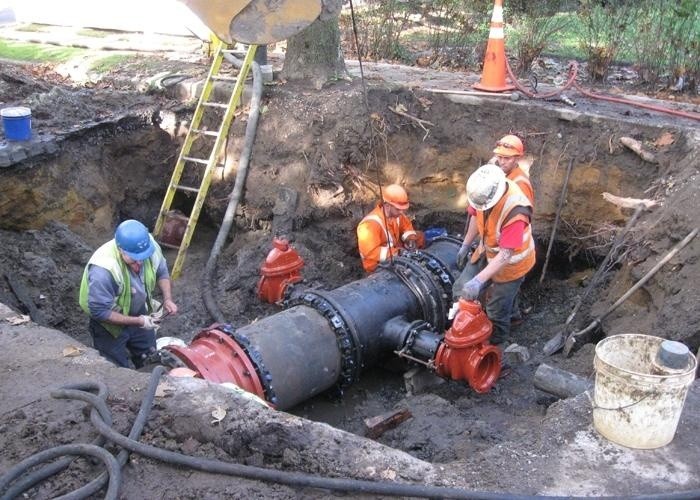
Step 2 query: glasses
495,140,521,153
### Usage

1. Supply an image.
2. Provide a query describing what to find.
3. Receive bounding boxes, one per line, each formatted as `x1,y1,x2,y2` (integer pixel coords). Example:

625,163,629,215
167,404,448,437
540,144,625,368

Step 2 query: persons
357,184,425,275
454,165,538,379
78,218,178,370
485,134,535,330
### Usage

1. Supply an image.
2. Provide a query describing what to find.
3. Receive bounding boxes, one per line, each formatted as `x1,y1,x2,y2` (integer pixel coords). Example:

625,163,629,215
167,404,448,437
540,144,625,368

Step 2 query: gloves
138,314,161,331
461,277,485,301
405,239,416,250
399,249,410,259
456,244,470,271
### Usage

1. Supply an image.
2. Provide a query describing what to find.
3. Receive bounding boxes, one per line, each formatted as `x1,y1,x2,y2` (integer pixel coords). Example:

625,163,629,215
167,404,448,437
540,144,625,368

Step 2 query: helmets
114,218,155,261
465,164,506,211
493,133,525,158
382,184,410,210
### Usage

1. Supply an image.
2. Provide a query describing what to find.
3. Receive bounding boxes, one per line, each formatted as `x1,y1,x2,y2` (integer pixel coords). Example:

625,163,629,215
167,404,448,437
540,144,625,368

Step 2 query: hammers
421,87,522,101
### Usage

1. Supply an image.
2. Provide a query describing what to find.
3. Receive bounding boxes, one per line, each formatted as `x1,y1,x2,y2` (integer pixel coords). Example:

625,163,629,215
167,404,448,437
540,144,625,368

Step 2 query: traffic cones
472,1,516,95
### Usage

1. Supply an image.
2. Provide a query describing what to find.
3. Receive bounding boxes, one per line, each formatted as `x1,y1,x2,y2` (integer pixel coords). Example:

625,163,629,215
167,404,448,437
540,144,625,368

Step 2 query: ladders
152,43,257,280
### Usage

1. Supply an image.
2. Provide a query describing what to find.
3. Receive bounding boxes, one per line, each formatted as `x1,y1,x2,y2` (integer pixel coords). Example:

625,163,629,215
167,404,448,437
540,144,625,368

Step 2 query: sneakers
510,310,523,328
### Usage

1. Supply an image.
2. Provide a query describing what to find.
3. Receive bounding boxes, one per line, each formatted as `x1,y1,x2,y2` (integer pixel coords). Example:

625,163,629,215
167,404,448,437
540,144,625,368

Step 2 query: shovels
544,205,698,360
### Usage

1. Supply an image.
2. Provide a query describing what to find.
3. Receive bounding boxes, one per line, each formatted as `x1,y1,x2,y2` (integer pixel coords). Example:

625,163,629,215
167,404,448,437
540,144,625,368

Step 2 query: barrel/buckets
0,107,32,142
594,333,697,450
